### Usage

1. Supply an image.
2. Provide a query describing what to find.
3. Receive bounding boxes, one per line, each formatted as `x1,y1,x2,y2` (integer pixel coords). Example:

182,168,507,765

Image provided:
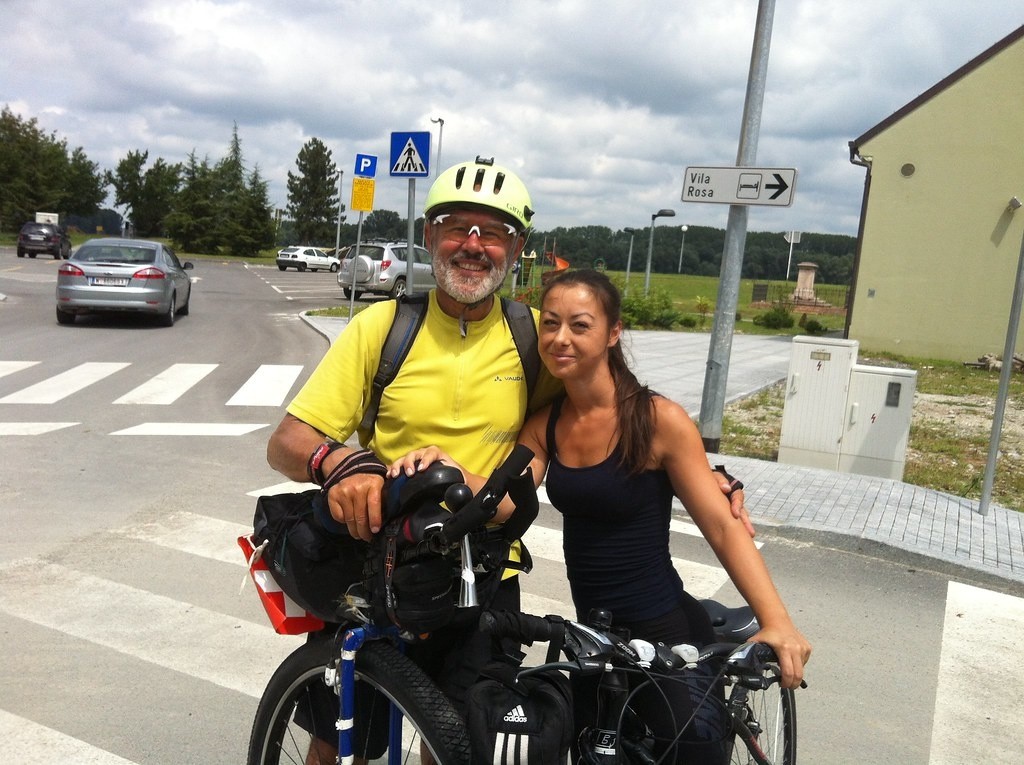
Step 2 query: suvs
17,221,73,260
336,237,438,303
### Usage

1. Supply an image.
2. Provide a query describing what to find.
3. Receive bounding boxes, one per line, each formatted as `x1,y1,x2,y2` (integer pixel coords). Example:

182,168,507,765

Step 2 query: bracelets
307,441,348,487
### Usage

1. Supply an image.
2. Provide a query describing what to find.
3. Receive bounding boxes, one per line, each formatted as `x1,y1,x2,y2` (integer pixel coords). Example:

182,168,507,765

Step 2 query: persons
386,271,812,764
266,155,756,765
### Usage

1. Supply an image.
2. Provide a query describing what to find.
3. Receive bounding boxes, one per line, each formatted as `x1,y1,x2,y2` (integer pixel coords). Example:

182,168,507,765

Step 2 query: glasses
433,214,517,246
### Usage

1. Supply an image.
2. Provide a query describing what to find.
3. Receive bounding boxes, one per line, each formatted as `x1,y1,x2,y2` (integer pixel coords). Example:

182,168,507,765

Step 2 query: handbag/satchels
238,487,517,634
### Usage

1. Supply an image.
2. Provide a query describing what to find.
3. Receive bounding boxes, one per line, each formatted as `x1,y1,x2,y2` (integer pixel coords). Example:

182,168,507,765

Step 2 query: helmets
423,155,535,234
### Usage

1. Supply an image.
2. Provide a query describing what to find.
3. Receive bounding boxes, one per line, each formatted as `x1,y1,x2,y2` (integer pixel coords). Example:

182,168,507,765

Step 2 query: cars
55,237,194,327
276,245,341,272
323,246,348,264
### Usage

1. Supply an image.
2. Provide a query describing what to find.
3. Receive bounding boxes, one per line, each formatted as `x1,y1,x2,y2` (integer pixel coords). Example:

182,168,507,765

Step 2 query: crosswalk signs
390,131,433,178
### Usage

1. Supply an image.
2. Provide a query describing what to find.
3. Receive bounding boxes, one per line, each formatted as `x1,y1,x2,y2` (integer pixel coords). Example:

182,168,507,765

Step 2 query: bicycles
246,442,810,765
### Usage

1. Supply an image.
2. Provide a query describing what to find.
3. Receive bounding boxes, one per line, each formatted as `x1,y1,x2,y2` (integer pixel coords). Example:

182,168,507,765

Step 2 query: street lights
642,210,678,297
429,118,445,182
623,227,637,298
677,226,689,274
334,168,343,258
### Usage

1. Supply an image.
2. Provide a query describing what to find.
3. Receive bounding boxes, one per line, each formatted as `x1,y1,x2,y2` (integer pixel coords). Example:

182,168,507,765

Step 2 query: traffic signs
681,165,798,208
